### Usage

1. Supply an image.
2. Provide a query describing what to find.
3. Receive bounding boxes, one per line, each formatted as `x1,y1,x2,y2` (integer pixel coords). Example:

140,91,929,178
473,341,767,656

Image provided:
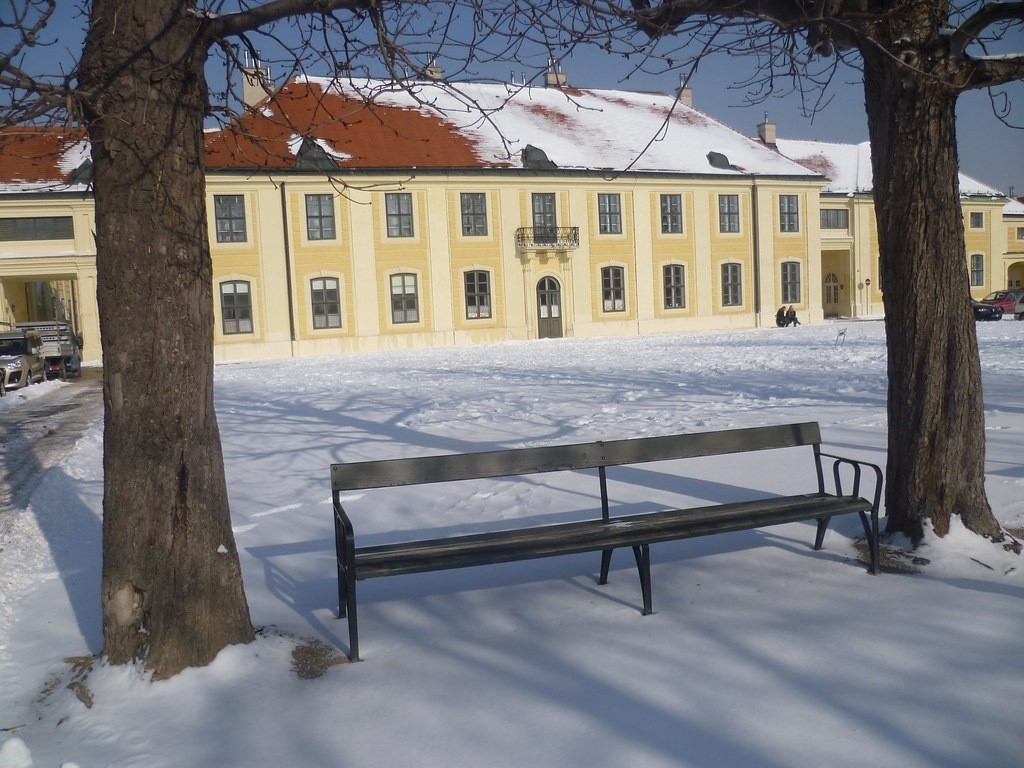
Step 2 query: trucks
20,318,84,381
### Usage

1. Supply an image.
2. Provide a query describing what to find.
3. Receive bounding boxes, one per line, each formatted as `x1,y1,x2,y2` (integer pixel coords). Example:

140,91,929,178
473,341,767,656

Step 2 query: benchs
330,423,889,662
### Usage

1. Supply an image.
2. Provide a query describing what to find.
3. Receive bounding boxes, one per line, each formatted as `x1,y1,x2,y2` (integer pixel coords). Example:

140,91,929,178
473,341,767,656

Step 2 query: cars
971,297,1004,320
979,290,1016,314
1012,294,1024,322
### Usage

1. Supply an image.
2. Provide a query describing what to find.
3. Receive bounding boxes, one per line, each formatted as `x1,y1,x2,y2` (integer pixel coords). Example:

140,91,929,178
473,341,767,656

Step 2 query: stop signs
865,279,870,286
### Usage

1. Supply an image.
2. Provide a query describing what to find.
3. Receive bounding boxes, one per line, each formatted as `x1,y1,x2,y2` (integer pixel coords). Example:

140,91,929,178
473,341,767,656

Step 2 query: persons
786,305,802,327
776,306,788,327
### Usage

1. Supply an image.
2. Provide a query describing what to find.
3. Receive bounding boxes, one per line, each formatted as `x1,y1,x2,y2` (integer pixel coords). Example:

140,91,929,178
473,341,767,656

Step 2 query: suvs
0,329,47,395
